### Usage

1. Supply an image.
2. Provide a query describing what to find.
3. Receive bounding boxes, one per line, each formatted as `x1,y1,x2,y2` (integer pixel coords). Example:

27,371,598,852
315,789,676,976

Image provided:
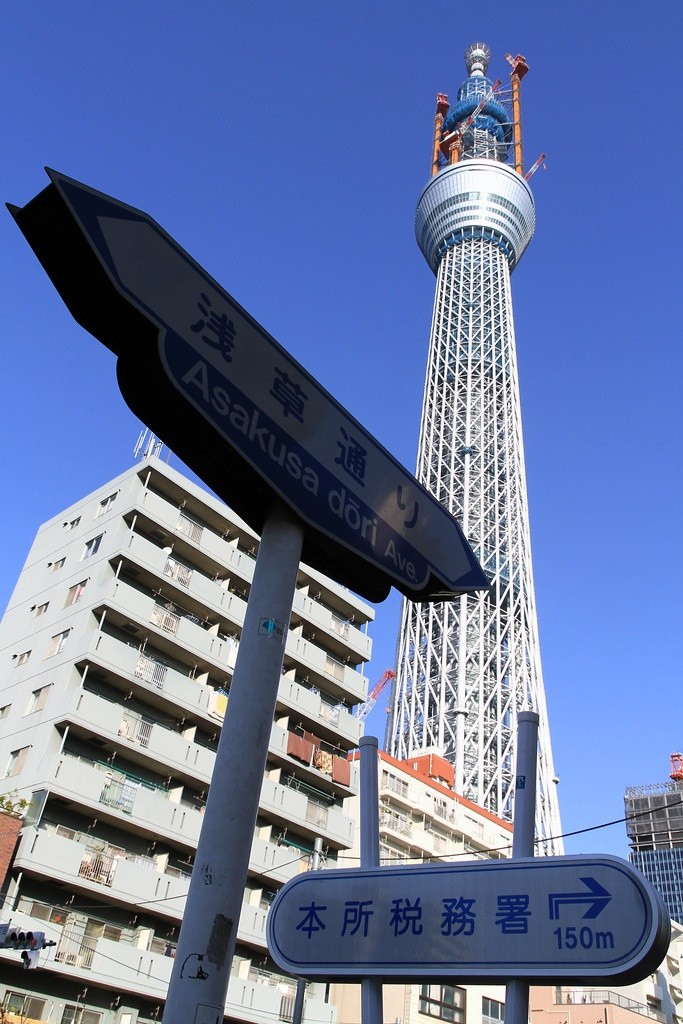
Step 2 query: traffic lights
10,930,45,948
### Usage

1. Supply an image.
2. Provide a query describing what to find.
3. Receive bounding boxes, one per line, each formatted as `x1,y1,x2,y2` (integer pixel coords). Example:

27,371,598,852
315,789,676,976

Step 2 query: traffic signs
265,853,672,988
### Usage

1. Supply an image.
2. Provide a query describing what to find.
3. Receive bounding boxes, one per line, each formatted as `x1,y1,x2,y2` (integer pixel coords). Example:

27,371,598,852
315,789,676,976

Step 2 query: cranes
353,668,396,722
